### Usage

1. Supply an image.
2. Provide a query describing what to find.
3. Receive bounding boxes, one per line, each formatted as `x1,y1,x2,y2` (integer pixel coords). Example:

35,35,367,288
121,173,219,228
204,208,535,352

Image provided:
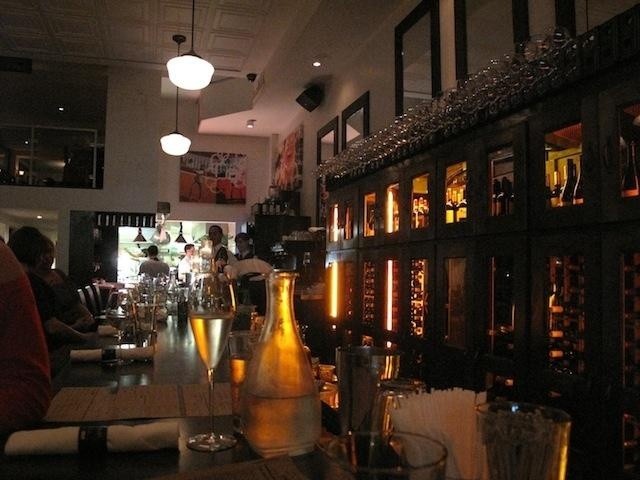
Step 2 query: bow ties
239,254,243,260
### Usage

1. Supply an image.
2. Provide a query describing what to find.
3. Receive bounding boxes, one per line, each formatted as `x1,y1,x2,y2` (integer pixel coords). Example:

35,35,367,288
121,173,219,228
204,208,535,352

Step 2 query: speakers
295,85,323,112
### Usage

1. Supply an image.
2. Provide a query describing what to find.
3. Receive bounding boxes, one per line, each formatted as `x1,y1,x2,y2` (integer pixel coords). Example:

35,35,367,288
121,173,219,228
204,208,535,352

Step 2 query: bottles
242,271,321,458
447,186,469,223
413,197,430,228
544,155,584,207
621,141,640,198
491,175,514,217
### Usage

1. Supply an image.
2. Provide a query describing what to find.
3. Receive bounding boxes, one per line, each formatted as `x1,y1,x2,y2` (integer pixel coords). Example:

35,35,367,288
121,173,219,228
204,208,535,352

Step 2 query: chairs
78,281,124,328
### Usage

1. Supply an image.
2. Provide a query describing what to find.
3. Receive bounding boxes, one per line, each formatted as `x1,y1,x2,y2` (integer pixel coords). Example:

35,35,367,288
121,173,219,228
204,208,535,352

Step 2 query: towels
68,343,157,362
5,421,180,455
97,322,155,334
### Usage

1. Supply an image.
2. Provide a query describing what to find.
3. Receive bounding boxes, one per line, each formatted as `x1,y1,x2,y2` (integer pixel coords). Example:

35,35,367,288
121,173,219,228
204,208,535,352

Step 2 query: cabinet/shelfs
241,215,312,263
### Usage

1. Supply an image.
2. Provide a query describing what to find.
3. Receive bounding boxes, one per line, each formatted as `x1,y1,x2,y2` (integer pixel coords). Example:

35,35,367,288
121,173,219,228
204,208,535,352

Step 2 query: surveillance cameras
247,73,257,81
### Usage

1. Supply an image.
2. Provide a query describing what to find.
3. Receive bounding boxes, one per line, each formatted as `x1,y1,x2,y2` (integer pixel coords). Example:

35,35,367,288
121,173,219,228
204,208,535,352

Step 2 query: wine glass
185,278,238,451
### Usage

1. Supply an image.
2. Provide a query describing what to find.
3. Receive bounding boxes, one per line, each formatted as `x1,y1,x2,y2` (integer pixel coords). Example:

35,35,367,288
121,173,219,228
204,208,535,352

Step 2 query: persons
233,233,253,261
0,226,97,431
178,244,195,279
209,226,238,273
139,246,169,277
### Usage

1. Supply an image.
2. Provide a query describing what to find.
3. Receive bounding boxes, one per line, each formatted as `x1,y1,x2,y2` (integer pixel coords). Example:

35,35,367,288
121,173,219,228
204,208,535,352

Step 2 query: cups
334,343,447,479
545,302,586,362
471,402,570,479
104,272,179,367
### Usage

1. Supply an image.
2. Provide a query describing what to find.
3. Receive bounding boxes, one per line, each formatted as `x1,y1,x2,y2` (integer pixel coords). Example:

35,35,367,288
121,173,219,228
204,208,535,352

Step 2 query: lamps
246,120,254,130
175,222,188,243
133,227,147,242
157,34,193,157
166,1,215,90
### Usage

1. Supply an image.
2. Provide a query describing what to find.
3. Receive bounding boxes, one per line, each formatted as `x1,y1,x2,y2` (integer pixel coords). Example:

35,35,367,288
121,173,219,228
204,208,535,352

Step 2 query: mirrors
393,0,441,120
553,1,639,42
451,1,531,83
315,116,339,167
